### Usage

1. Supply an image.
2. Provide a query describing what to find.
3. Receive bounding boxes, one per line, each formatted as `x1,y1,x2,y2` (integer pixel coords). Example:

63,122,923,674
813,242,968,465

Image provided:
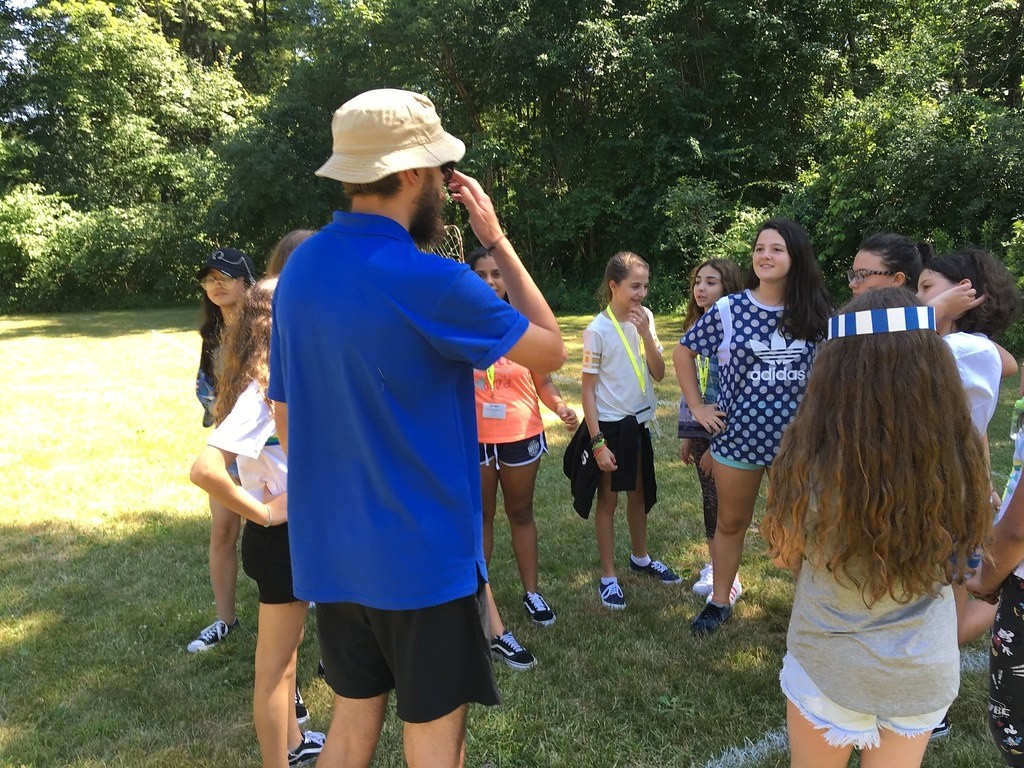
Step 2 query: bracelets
263,506,272,527
590,432,607,457
487,234,505,255
554,401,567,410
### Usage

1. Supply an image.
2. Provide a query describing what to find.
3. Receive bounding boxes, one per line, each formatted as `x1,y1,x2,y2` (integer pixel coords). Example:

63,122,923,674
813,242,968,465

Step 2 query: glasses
201,276,236,290
847,268,911,284
440,162,454,180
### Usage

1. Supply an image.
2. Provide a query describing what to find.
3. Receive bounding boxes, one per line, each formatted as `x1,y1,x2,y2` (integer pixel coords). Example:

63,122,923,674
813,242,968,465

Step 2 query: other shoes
690,602,733,637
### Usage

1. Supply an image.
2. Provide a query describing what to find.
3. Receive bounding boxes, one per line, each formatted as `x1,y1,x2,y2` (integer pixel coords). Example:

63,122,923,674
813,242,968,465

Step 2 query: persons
672,220,835,638
486,582,537,670
267,88,566,768
847,235,932,294
581,250,681,611
191,273,326,768
964,367,1024,768
677,259,742,606
186,247,259,654
760,286,998,768
915,252,1017,736
268,230,327,721
466,249,579,624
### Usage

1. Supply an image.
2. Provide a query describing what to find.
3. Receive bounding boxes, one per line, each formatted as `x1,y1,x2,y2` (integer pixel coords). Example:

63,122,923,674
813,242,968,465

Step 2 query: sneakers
490,630,538,669
523,591,557,627
929,718,950,738
186,615,240,654
629,557,682,585
706,573,742,606
599,582,626,609
294,680,310,724
286,730,326,768
692,564,714,595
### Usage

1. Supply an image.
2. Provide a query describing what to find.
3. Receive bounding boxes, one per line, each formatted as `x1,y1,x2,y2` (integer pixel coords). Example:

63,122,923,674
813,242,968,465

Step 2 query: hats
197,249,255,280
314,89,465,184
